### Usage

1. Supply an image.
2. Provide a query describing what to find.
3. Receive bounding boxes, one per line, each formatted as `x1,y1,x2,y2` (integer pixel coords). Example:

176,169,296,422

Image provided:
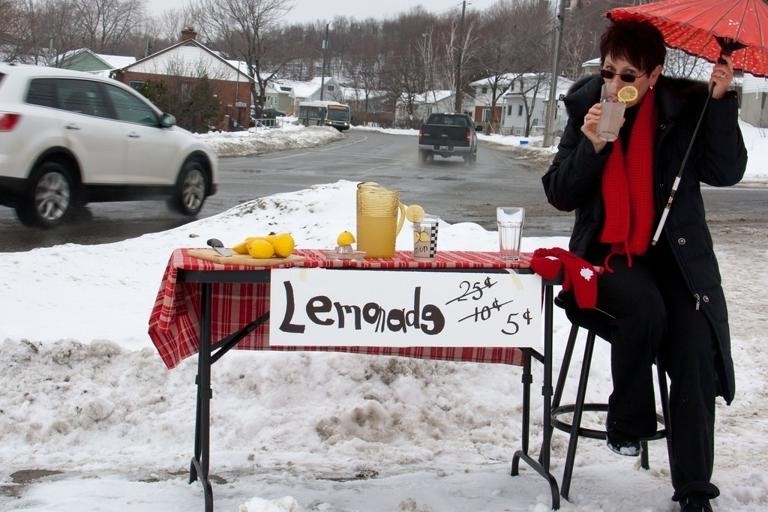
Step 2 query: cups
493,204,526,260
355,183,406,258
411,211,439,262
596,82,628,144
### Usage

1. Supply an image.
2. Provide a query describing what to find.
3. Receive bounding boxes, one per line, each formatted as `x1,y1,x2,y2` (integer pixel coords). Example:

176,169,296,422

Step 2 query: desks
174,248,609,512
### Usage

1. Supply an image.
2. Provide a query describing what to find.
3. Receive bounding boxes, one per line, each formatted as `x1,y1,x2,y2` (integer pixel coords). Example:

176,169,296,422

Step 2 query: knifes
207,237,232,257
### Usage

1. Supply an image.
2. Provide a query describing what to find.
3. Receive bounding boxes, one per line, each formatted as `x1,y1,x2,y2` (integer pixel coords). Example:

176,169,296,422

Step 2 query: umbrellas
599,0,768,245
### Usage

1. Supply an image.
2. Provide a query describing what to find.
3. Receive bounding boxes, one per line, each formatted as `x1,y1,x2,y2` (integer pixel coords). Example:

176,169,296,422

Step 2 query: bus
298,100,351,132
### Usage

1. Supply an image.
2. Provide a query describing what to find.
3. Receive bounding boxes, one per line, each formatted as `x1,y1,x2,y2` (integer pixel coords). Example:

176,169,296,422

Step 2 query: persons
539,17,747,511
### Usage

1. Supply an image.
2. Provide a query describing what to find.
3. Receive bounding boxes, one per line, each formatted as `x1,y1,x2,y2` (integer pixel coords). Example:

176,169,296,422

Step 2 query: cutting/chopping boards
191,248,308,268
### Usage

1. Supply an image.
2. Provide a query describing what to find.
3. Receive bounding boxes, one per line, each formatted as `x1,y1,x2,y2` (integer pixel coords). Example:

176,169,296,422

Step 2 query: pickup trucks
418,112,477,165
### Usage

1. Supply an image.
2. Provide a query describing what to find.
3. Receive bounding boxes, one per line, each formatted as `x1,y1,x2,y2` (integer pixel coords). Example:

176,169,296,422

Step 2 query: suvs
0,63,219,231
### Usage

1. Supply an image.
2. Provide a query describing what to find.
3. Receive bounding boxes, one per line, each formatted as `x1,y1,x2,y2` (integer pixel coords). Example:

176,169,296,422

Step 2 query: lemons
406,205,424,223
232,233,294,259
337,230,356,246
618,86,638,103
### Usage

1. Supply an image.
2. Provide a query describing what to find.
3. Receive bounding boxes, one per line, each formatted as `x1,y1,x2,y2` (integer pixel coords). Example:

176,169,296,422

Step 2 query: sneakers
607,417,640,457
680,492,713,512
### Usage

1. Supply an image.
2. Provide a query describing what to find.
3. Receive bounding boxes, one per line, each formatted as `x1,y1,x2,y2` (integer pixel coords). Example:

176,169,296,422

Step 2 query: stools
537,275,678,498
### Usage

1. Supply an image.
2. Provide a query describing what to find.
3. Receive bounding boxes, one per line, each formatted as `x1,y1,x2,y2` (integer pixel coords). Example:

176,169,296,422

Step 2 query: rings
720,72,727,78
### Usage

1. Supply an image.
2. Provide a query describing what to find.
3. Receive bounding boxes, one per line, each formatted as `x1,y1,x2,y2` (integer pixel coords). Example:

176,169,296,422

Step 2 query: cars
262,108,286,118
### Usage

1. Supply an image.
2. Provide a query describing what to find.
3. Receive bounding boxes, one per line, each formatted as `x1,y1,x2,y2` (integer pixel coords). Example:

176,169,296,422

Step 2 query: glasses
599,61,650,82
